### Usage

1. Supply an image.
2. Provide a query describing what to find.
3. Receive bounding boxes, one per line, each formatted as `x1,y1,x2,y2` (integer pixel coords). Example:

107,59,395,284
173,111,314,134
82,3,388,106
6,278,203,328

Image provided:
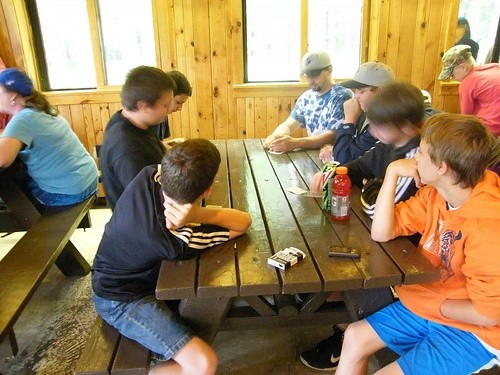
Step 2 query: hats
438,44,472,80
300,50,331,77
0,69,33,95
340,61,395,89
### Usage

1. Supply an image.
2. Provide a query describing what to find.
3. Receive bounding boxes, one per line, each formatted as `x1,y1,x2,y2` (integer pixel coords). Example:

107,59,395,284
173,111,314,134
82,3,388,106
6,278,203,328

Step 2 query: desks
155,138,441,370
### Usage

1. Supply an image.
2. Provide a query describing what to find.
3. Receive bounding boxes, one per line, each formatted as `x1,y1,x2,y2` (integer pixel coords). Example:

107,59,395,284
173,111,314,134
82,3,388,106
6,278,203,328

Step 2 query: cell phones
328,246,361,258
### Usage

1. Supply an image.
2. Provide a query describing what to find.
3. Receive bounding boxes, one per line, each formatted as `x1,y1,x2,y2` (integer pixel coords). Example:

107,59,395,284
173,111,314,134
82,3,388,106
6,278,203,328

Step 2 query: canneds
322,161,341,212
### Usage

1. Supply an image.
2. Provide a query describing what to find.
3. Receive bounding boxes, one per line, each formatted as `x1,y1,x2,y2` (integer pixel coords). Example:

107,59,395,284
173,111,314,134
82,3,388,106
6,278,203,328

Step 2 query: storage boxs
268,247,306,270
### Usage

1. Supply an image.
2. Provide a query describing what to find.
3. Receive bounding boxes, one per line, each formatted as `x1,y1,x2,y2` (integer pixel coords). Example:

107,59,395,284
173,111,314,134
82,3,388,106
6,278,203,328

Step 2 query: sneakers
299,324,345,371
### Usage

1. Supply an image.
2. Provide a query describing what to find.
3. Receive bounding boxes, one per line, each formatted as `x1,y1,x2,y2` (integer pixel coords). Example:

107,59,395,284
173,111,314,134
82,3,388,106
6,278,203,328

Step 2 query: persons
150,70,192,142
438,44,500,139
0,68,99,209
420,90,443,121
334,112,500,375
457,17,479,61
89,138,250,375
318,60,400,164
99,65,175,213
300,80,427,370
263,49,354,153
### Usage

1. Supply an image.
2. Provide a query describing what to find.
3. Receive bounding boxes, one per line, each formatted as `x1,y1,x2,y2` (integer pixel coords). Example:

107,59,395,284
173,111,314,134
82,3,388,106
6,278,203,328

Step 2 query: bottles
330,166,351,222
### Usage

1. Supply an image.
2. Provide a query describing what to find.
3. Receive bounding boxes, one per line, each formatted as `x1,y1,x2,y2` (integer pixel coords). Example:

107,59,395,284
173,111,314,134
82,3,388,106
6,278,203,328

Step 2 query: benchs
76,313,151,375
0,194,95,359
